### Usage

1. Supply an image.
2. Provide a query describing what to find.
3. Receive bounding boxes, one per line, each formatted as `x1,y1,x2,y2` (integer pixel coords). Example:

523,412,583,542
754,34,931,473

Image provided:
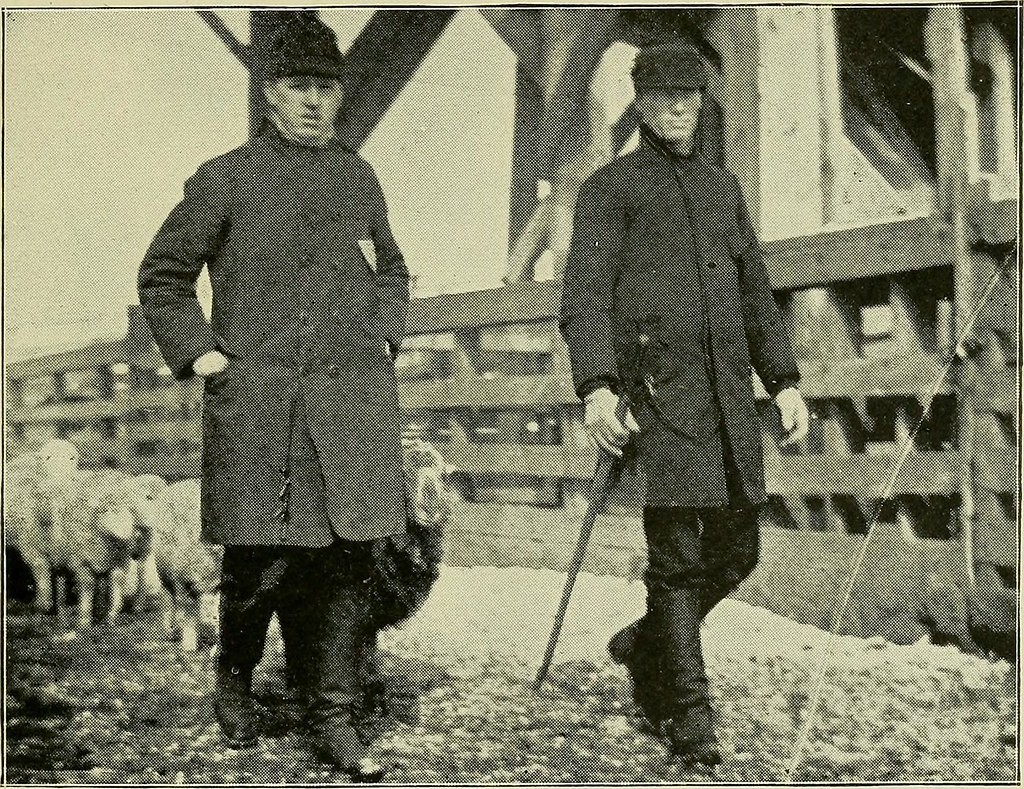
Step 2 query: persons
559,45,810,768
137,16,410,782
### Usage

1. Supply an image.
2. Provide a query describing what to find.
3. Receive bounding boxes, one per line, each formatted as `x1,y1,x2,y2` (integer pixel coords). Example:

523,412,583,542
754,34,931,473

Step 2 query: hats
260,15,345,78
631,42,707,88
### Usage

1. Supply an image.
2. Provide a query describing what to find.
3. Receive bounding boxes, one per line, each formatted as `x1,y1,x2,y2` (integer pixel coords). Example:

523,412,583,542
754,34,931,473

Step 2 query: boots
303,587,385,782
660,588,711,764
214,599,274,745
608,612,670,733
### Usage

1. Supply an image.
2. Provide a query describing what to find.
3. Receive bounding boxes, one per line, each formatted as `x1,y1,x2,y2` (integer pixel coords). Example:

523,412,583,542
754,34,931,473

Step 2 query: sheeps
3,436,458,709
